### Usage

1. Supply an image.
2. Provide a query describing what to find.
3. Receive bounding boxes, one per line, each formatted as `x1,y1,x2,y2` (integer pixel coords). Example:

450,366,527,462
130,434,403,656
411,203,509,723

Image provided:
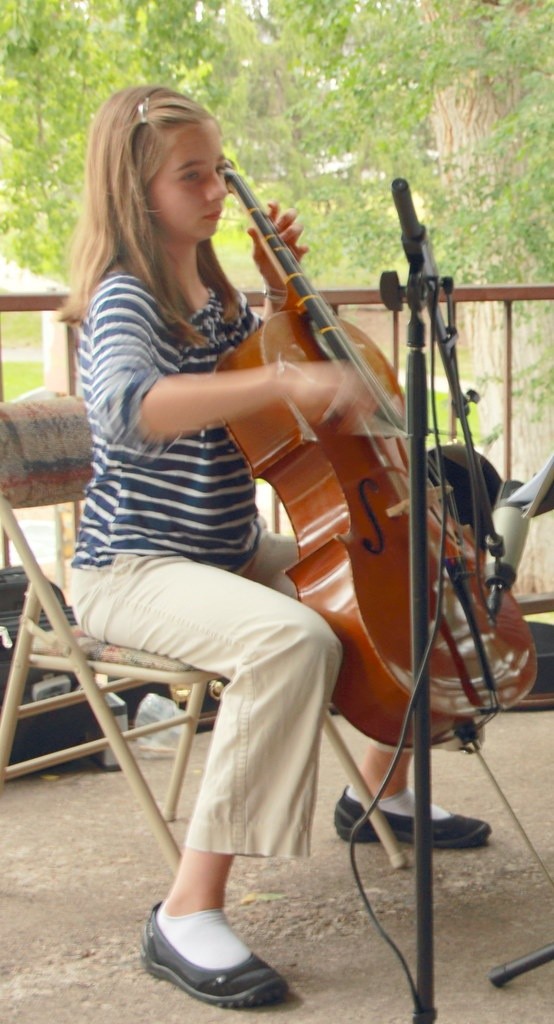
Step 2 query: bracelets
262,286,289,305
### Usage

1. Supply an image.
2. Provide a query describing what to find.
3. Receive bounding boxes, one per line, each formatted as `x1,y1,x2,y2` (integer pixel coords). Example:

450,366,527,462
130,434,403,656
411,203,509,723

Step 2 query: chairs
0,389,411,891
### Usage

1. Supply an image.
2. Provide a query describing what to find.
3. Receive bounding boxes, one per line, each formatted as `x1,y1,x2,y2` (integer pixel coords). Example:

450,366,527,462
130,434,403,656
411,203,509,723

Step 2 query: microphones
483,479,531,623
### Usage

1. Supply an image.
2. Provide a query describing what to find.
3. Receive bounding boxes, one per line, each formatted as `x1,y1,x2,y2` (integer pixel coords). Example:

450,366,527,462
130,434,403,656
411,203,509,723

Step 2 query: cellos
214,147,538,751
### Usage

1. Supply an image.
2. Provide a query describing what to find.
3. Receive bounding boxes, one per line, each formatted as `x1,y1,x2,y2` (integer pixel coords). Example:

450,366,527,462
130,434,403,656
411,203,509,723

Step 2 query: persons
55,84,495,1011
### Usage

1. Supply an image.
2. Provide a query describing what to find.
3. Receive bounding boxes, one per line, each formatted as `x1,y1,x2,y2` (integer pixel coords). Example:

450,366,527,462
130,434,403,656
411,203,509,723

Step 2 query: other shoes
333,786,486,848
130,901,289,1008
